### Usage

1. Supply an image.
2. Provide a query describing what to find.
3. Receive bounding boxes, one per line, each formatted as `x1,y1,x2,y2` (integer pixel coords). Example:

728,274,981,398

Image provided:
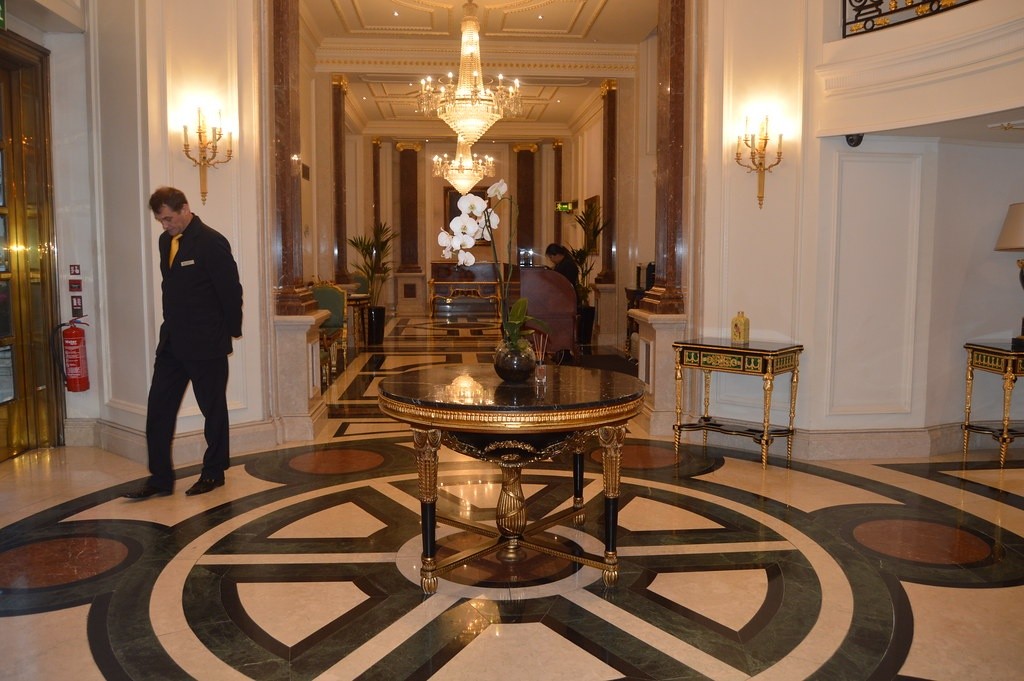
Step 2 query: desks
378,365,644,596
672,336,804,470
961,344,1024,468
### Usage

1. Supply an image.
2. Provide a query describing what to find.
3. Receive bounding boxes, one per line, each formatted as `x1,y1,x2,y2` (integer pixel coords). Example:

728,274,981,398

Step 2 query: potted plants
569,204,606,345
346,221,398,345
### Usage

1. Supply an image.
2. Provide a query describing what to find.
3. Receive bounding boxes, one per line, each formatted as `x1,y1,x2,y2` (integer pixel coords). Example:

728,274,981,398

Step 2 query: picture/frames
582,196,600,257
442,186,493,247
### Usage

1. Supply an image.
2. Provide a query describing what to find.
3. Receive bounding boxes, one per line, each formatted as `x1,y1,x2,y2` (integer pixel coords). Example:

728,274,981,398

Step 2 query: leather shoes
123,474,176,498
185,470,226,496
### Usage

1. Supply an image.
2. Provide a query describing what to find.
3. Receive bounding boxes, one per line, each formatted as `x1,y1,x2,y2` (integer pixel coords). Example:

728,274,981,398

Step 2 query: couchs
429,261,501,319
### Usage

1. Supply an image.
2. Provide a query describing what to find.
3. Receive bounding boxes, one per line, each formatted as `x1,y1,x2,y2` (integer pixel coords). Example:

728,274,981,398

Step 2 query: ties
169,233,183,269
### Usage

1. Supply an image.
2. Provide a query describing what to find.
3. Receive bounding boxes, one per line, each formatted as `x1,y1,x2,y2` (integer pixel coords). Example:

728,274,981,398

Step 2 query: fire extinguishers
50,314,91,392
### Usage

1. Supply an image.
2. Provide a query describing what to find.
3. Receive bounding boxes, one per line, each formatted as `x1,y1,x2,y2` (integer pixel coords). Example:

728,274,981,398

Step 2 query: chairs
311,280,348,371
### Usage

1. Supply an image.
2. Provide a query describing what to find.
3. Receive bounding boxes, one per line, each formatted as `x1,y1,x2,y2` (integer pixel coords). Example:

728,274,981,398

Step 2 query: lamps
435,135,496,193
289,154,301,175
165,75,239,203
735,113,783,208
415,2,521,148
993,201,1024,352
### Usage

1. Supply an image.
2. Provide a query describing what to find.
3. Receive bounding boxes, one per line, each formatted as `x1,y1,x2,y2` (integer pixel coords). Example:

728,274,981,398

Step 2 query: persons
120,187,243,499
545,243,579,287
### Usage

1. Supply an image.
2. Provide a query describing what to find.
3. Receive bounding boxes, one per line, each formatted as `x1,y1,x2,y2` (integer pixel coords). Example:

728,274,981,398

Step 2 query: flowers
438,180,549,336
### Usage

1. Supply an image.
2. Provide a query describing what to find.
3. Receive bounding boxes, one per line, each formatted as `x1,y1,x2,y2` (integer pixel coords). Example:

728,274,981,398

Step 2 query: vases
494,339,536,384
